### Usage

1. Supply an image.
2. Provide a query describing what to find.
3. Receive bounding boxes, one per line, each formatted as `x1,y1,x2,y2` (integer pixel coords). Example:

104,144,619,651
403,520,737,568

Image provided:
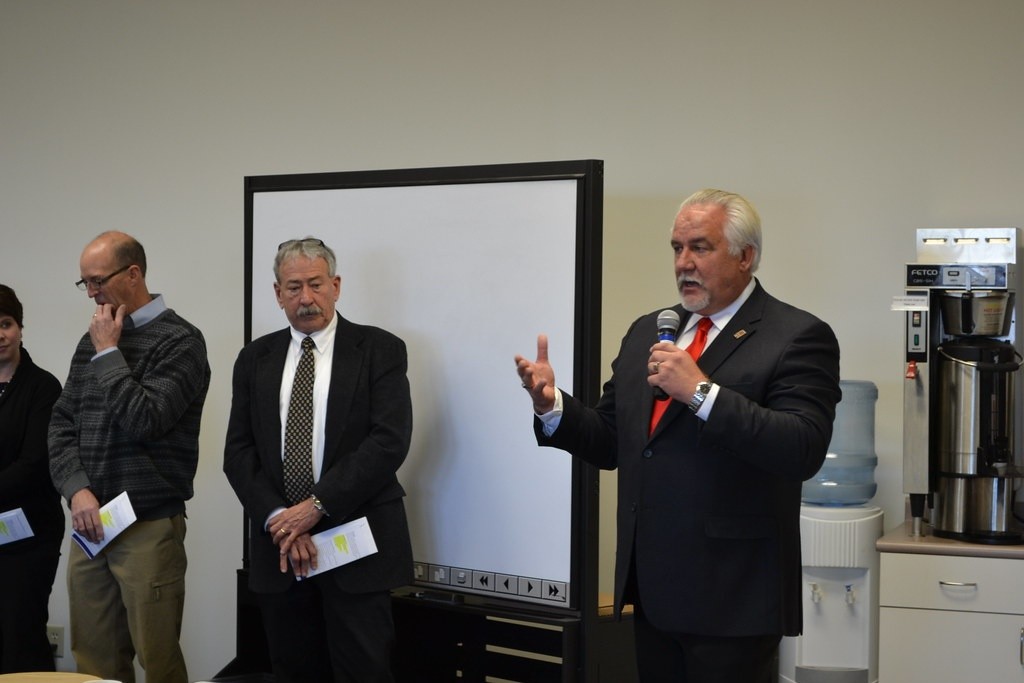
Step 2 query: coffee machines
901,228,1024,544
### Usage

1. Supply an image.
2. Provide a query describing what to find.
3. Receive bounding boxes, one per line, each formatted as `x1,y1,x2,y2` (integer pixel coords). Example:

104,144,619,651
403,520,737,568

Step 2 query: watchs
310,493,330,516
689,380,713,413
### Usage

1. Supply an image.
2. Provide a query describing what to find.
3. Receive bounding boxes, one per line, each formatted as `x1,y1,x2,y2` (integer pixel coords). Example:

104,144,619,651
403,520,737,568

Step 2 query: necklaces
0,371,14,397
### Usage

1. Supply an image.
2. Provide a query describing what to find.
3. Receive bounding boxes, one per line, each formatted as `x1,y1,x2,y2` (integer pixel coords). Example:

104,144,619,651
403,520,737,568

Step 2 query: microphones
653,309,680,401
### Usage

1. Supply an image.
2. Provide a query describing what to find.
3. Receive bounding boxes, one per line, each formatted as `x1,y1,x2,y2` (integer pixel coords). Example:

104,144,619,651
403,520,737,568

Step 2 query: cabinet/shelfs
876,496,1024,683
236,568,637,683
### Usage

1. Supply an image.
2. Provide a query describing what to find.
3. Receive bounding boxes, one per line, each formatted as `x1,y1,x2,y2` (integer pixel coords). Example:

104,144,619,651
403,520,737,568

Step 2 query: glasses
76,264,131,291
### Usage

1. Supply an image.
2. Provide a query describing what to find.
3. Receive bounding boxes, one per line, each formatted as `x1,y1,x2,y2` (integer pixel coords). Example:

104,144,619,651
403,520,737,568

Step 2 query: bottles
802,379,878,508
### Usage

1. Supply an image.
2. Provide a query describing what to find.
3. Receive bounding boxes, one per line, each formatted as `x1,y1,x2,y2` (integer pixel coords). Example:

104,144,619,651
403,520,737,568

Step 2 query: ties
649,316,715,435
285,337,317,507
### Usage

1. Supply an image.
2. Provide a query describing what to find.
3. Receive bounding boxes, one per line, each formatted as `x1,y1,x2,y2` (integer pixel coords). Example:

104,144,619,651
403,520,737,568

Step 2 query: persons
0,283,64,674
513,190,842,683
46,232,209,683
224,236,416,661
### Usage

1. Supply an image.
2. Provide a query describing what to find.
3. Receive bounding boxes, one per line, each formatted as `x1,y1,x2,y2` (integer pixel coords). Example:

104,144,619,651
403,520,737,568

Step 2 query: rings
93,314,97,317
281,528,286,534
522,382,535,388
653,361,663,373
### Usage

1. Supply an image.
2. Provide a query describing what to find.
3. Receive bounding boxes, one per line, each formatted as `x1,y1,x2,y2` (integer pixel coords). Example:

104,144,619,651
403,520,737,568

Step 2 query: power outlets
46,625,65,657
